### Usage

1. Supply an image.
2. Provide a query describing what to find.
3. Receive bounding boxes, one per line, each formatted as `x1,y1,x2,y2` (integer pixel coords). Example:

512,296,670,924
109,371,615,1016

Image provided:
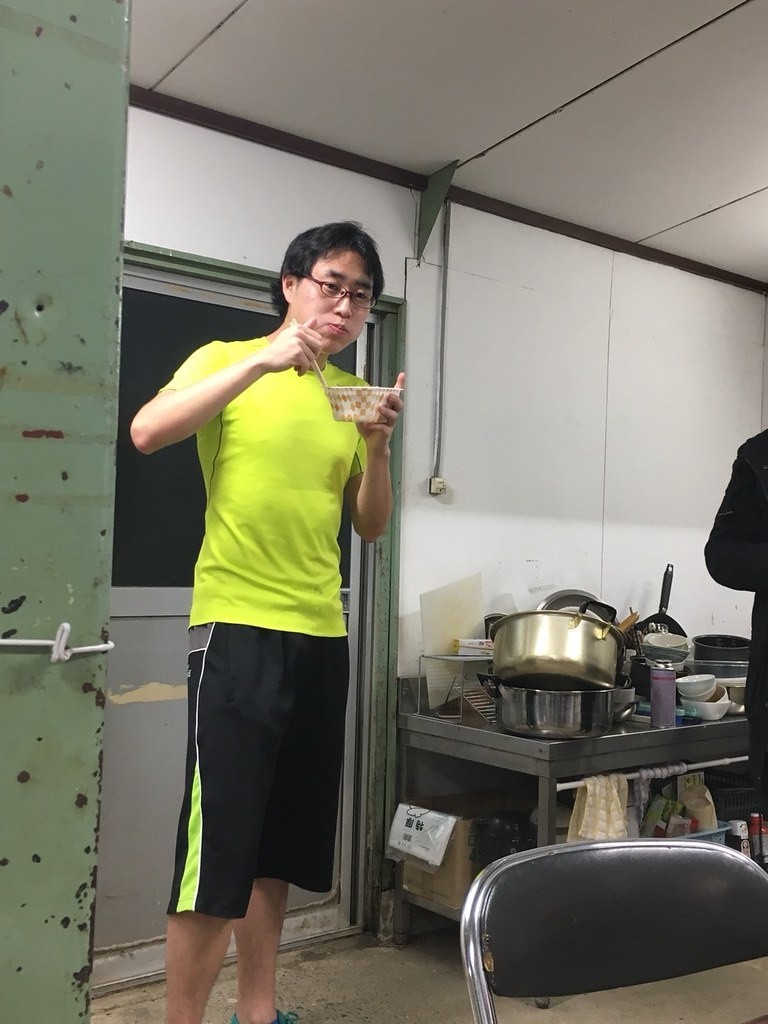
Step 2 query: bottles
748,813,768,874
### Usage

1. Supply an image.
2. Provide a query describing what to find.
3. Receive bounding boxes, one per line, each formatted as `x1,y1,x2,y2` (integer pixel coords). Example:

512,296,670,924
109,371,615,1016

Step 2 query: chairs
458,837,768,1024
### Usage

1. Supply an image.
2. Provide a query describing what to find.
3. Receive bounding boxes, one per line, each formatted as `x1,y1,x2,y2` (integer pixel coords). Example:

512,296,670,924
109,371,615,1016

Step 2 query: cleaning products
727,812,768,865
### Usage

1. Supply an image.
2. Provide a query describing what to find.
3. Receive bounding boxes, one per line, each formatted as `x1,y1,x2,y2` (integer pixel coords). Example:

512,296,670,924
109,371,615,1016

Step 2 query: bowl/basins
677,674,731,720
613,701,640,723
642,633,690,672
325,387,403,423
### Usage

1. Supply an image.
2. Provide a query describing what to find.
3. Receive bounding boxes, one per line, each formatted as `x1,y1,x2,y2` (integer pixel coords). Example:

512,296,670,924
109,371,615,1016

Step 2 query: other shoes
231,1009,300,1024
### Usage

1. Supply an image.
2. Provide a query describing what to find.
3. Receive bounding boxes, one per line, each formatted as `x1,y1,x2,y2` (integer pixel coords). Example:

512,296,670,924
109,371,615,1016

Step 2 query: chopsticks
290,319,327,386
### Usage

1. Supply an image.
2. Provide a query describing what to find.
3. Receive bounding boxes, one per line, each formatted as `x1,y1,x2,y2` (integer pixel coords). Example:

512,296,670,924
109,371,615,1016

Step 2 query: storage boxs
401,790,538,912
650,759,761,824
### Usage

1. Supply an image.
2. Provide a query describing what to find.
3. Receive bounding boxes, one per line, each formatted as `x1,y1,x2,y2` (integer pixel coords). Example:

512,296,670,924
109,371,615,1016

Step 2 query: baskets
672,820,732,845
703,768,761,825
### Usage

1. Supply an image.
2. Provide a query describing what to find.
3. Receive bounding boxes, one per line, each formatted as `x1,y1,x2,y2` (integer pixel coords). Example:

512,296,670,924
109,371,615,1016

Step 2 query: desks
399,709,768,945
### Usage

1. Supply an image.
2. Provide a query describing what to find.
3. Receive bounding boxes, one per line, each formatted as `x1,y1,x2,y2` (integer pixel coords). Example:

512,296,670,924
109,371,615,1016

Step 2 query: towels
633,761,687,828
565,772,627,841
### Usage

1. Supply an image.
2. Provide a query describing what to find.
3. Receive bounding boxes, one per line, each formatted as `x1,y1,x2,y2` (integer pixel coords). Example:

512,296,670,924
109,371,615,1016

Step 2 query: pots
490,600,625,692
477,673,631,739
632,564,687,651
630,656,691,705
693,635,750,661
693,660,749,678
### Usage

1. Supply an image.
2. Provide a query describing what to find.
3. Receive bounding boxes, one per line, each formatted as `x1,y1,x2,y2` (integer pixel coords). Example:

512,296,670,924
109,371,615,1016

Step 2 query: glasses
296,269,379,310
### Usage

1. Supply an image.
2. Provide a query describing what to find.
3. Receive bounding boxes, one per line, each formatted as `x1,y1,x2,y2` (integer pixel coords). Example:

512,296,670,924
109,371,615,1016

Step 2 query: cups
613,686,635,702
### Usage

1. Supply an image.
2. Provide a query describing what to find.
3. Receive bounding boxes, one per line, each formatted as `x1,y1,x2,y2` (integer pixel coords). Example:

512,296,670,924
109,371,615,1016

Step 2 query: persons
703,429,768,818
131,221,406,1024
